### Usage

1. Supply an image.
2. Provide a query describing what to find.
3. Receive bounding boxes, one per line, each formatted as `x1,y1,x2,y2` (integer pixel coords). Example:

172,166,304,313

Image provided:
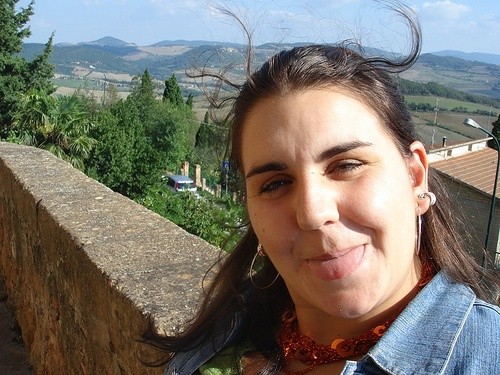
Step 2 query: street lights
463,117,500,282
101,72,106,103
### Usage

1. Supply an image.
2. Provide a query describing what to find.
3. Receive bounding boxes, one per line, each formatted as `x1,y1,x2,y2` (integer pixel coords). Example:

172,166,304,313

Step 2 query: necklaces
279,242,438,367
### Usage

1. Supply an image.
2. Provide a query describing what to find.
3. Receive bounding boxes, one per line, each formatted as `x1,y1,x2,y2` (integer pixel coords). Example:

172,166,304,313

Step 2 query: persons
132,0,499,375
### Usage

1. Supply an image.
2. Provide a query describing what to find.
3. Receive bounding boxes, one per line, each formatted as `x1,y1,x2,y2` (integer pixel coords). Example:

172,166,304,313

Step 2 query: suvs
157,175,197,193
